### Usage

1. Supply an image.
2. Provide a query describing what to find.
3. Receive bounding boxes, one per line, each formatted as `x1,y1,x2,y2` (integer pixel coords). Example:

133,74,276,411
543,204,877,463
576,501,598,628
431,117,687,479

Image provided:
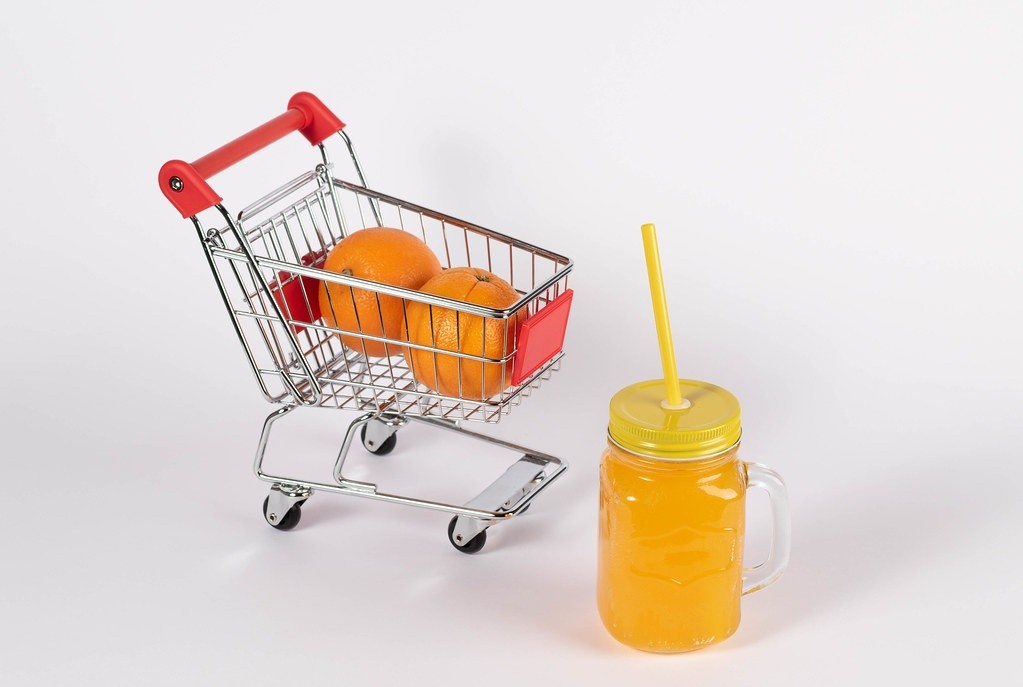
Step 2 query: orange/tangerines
402,267,530,399
319,227,441,358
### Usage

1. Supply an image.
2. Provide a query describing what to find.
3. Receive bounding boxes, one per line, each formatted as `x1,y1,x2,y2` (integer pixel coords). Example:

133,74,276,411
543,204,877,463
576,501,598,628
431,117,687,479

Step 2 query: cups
596,377,791,654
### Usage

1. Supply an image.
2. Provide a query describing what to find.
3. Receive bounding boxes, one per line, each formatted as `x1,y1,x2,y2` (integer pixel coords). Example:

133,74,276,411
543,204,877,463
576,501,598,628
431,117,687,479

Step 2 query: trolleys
156,90,573,554
